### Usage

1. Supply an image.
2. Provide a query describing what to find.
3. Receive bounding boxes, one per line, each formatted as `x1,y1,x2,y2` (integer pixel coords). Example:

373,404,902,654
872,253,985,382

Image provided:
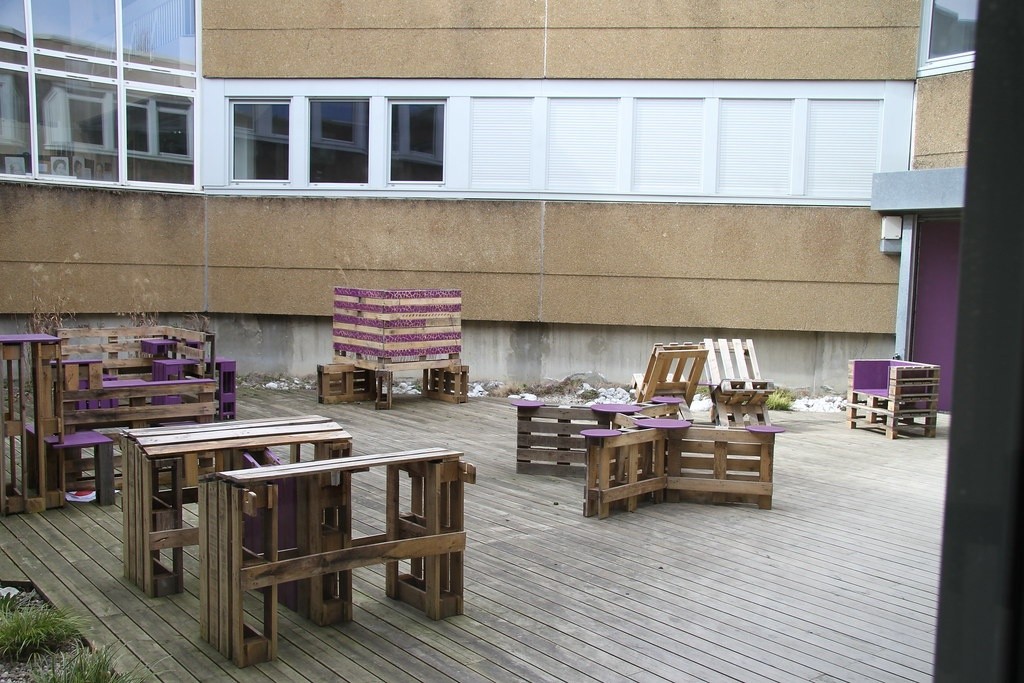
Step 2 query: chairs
632,338,776,428
847,358,941,440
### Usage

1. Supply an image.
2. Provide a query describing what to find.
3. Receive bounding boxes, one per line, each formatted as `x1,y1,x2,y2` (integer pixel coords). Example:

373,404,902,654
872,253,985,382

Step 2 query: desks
120,415,476,667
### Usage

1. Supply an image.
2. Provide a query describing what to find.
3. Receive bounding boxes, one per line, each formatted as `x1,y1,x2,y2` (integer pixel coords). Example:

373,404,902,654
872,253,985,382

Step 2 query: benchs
511,394,785,521
